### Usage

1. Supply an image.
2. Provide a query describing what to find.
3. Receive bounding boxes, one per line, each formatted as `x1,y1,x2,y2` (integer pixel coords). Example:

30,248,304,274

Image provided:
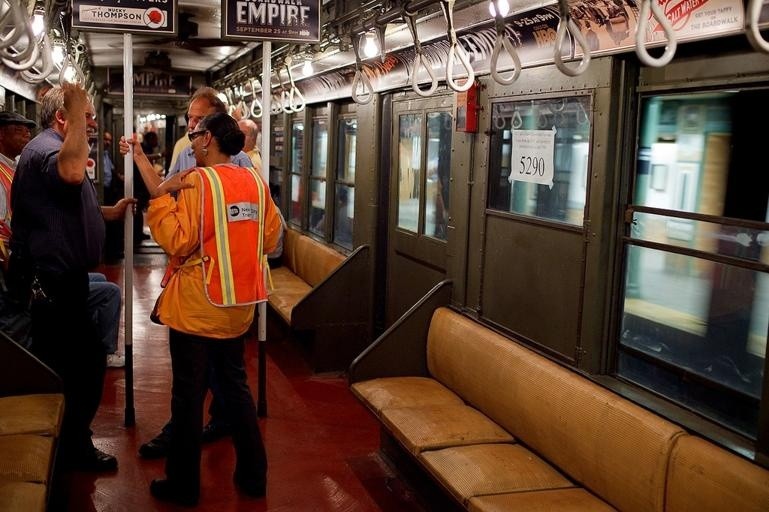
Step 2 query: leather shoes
138,428,177,458
201,416,230,444
142,233,150,240
58,446,117,472
149,479,198,507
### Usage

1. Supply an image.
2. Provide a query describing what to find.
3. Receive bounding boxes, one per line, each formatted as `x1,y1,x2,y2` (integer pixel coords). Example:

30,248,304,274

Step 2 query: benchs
0,328,67,510
268,225,371,374
348,279,769,511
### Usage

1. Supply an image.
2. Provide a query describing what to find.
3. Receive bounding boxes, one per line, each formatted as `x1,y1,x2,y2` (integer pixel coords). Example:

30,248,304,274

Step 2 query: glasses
188,130,207,142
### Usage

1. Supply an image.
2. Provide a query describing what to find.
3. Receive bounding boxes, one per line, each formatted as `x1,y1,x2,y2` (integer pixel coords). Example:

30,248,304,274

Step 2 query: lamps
364,29,379,58
487,0,511,19
30,9,44,36
61,60,76,80
50,36,64,63
302,53,314,76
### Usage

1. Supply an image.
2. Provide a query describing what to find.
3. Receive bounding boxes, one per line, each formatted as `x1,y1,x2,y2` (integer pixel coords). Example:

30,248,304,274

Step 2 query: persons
120,85,289,508
9,82,138,511
1,110,124,473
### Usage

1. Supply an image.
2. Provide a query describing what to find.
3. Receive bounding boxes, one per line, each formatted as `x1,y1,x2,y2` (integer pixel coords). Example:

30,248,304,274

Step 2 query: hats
0,111,36,128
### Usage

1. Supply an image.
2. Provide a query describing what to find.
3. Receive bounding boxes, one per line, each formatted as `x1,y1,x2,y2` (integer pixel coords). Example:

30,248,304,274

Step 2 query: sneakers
106,351,125,368
